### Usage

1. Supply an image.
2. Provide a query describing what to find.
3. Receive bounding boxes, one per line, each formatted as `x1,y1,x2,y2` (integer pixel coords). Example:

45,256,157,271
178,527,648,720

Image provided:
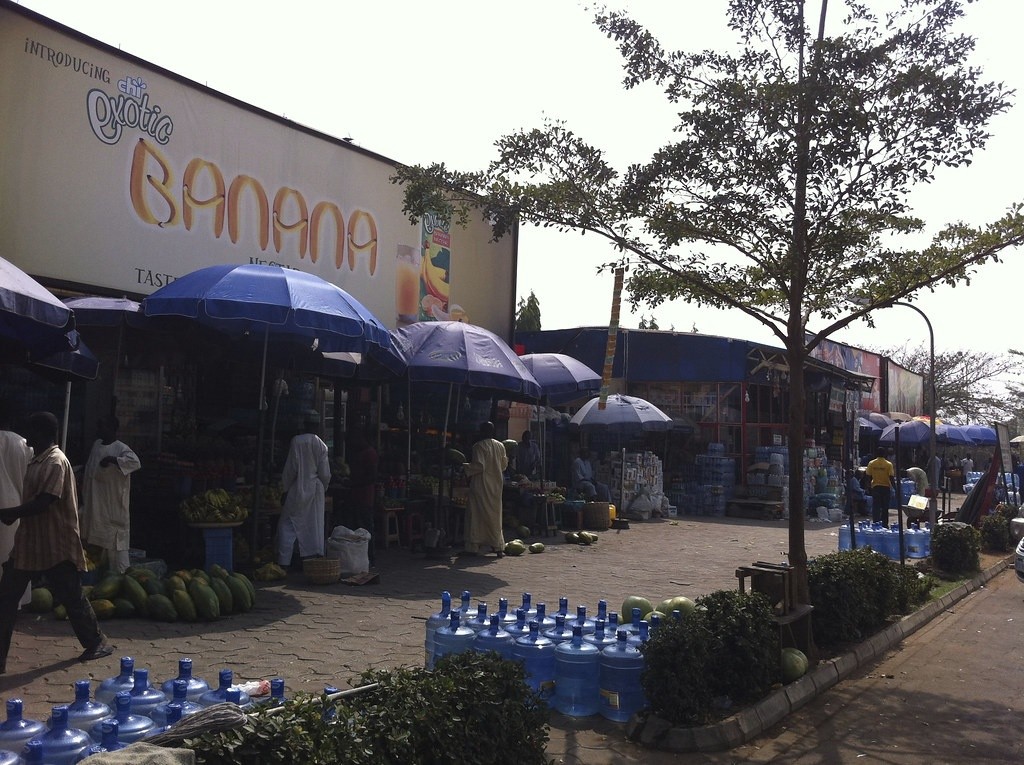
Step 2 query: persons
568,449,612,502
925,454,942,494
77,414,141,574
984,458,993,471
900,467,929,497
0,411,114,674
457,422,508,559
0,431,34,609
517,430,542,478
865,446,897,530
847,470,868,515
275,410,333,568
347,432,380,543
960,453,975,482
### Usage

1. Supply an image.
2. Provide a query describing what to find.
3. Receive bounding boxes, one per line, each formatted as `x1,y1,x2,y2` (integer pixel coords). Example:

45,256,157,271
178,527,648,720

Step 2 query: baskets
303,558,340,584
584,501,609,529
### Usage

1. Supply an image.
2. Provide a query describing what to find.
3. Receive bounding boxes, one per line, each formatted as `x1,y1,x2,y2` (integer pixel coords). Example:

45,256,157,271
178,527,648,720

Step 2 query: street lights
848,295,938,535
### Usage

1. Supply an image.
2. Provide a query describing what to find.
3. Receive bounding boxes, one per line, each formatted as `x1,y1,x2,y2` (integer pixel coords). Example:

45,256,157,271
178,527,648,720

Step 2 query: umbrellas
858,411,999,465
0,255,671,534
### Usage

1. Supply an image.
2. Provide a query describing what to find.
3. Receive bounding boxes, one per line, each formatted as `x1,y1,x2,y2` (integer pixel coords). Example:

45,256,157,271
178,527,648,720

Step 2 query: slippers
81,646,114,659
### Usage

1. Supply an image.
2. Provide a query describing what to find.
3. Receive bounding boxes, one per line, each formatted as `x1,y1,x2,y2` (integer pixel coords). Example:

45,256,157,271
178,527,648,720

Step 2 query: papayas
31,563,255,621
502,517,598,555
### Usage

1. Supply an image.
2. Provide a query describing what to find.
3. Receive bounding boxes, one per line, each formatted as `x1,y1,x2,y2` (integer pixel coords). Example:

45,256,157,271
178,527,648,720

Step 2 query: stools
534,501,557,536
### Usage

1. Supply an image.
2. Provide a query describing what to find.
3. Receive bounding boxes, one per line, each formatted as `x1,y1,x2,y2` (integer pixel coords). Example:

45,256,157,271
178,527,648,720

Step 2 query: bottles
664,443,736,517
0,657,338,765
746,446,839,518
839,519,931,561
890,477,916,506
425,591,680,723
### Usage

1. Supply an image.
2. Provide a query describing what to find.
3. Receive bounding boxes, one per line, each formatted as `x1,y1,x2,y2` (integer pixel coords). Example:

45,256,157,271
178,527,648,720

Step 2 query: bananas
178,486,247,523
257,562,282,581
421,241,449,302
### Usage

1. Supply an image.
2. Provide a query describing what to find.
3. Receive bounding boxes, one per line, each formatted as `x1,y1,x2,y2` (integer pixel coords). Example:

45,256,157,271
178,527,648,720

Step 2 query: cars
1013,536,1024,583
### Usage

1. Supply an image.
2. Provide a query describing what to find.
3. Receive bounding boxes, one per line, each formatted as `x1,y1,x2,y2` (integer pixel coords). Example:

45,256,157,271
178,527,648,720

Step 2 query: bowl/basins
612,519,629,526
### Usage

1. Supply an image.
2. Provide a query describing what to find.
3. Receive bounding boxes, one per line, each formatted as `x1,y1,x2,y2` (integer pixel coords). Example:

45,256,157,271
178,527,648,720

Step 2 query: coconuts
779,646,808,683
610,596,694,626
